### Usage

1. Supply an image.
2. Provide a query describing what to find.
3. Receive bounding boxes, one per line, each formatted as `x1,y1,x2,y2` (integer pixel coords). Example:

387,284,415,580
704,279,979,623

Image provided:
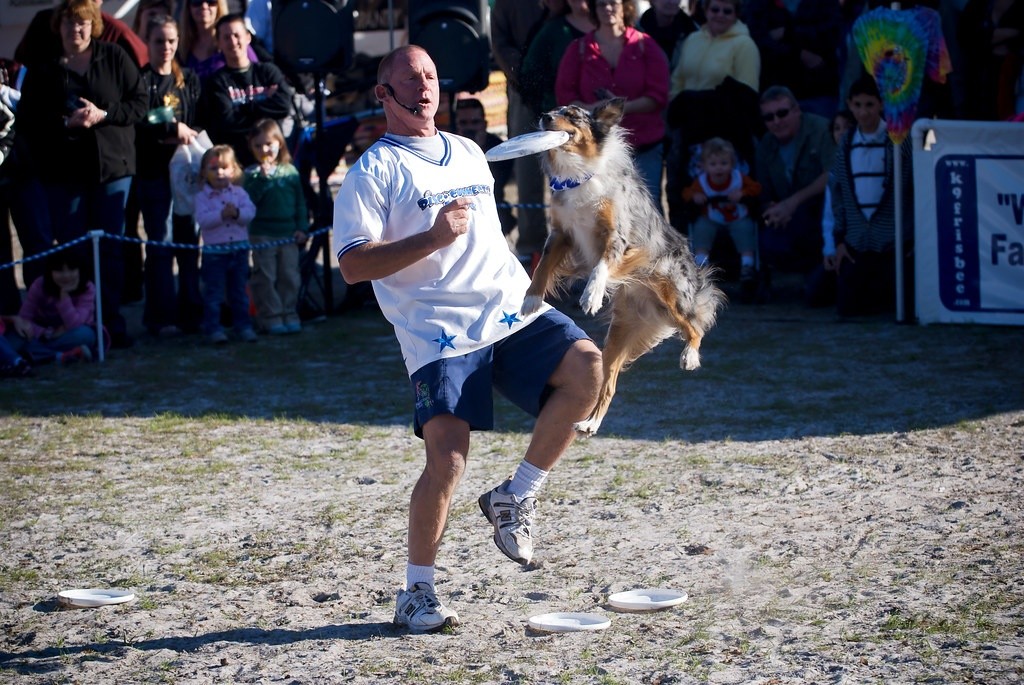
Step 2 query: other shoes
284,320,301,332
240,328,258,341
210,331,227,343
741,263,756,276
265,323,287,333
0,360,34,378
62,344,93,365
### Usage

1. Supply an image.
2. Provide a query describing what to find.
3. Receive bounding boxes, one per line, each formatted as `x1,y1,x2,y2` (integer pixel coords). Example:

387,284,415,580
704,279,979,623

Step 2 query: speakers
406,0,491,95
272,0,355,74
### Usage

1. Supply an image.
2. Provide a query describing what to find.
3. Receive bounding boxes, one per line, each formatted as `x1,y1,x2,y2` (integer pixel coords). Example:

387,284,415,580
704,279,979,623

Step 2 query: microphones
383,83,417,116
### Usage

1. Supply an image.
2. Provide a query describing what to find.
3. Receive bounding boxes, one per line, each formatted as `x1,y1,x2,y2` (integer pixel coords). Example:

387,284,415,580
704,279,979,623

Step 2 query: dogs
520,97,729,436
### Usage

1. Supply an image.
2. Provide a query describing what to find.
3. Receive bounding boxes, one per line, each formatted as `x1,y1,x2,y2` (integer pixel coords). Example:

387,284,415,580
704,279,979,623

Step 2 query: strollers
656,86,776,309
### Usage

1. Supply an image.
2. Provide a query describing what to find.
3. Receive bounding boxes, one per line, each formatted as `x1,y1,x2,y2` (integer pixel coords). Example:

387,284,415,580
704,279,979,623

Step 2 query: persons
334,46,604,634
0,0,1024,366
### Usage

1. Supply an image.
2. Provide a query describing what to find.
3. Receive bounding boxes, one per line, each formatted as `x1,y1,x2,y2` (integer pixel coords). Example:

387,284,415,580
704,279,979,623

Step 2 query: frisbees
527,611,612,632
484,130,570,163
607,588,688,610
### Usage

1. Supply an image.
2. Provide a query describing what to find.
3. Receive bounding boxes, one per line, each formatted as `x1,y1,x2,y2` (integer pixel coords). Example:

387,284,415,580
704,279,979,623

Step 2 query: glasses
708,6,736,16
762,106,793,122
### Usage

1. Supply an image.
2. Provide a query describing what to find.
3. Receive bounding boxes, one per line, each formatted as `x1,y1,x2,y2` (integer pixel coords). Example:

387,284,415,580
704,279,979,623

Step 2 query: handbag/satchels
169,130,217,216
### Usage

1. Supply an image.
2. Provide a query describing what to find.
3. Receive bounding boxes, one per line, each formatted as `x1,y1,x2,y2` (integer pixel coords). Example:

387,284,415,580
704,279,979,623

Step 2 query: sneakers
393,582,459,632
478,479,537,565
